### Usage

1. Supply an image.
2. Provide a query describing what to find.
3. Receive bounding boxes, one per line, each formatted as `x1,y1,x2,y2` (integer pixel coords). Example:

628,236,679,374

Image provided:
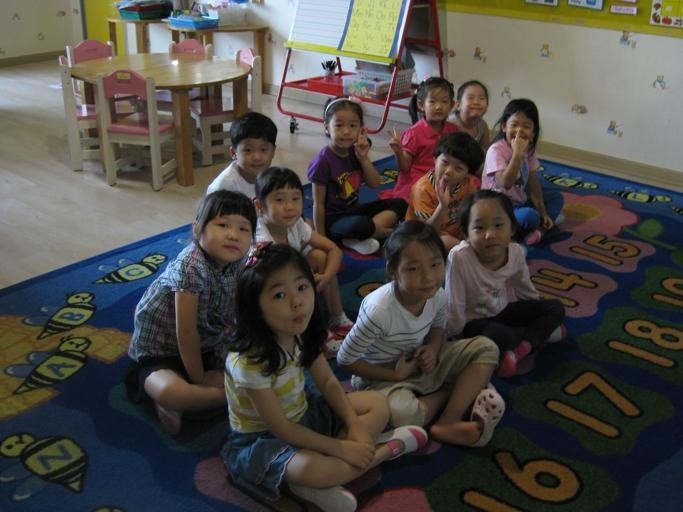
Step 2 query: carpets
0,159,682,511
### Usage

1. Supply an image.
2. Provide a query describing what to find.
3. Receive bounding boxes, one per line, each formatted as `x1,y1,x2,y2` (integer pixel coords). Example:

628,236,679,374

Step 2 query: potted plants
320,60,339,80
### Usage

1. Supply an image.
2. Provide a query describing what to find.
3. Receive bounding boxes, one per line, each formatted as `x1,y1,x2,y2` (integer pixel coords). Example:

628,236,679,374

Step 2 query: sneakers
287,482,358,512
326,315,357,337
316,331,345,359
342,234,381,256
375,424,431,462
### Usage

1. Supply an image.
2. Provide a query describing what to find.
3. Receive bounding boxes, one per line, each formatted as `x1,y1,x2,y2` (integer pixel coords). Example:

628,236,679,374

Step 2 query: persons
404,127,484,250
447,74,492,147
122,189,260,439
246,164,358,357
480,93,567,245
204,112,279,222
384,77,460,202
336,219,500,448
443,187,568,379
218,238,427,512
305,94,409,257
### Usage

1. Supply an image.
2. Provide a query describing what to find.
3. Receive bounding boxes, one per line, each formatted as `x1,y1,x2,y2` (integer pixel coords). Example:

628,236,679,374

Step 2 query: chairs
188,48,263,167
96,68,177,191
66,40,135,101
136,40,212,113
57,55,135,171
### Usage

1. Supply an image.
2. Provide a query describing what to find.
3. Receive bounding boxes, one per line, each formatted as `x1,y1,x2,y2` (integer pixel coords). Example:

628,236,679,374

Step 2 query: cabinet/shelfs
277,0,455,147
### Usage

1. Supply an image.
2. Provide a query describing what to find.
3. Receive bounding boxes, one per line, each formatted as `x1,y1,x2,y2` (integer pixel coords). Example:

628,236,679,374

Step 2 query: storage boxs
356,69,415,96
341,76,388,97
119,9,168,21
168,16,219,30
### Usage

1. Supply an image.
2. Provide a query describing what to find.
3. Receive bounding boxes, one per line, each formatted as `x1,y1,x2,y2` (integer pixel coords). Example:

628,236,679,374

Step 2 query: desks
166,22,269,94
71,53,251,187
106,15,179,53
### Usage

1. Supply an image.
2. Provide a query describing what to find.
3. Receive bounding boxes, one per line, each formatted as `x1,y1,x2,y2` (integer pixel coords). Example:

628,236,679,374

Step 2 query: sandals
467,388,504,450
